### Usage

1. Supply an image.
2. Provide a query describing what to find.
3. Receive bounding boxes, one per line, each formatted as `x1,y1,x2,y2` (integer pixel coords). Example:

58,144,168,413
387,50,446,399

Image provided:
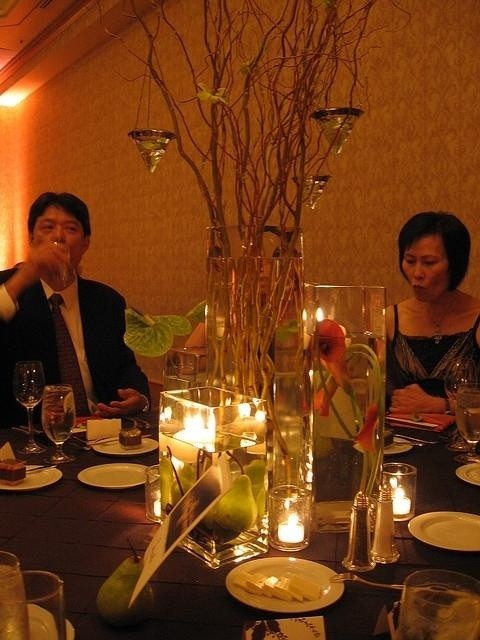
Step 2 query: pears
94,541,155,628
160,444,269,533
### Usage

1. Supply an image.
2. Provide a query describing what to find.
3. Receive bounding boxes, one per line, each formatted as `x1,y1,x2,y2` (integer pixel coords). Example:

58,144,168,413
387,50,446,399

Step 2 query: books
384,412,456,433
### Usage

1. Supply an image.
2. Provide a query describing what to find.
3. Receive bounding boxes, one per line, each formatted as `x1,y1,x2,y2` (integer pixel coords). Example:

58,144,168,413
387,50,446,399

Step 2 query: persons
0,192,152,431
179,226,302,375
368,210,480,413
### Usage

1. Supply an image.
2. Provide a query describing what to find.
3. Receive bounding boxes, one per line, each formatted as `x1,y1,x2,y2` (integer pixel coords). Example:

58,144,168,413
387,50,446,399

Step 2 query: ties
48,294,92,418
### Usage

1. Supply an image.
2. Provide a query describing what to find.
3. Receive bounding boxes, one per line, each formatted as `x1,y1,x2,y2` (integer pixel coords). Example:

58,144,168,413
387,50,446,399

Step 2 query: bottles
369,483,402,565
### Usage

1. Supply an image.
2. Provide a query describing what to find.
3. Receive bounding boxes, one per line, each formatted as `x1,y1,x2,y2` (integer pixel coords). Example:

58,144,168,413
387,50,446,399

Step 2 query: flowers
302,318,386,504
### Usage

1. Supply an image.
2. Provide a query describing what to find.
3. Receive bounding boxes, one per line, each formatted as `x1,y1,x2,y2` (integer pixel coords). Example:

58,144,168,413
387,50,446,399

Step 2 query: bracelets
141,393,150,413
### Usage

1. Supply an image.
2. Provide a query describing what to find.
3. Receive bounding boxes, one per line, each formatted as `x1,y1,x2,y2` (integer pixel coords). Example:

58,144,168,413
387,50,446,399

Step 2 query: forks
329,571,406,590
393,431,441,447
67,431,154,450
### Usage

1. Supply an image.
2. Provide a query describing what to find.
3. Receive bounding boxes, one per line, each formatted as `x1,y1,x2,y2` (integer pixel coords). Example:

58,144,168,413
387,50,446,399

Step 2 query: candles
273,497,307,546
385,484,413,516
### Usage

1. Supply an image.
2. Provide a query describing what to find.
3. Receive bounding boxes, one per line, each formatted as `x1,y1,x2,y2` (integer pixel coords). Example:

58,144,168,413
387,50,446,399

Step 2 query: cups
398,571,479,639
155,385,268,572
203,277,313,485
47,242,73,290
376,462,417,521
342,491,377,573
265,483,315,551
1,550,67,640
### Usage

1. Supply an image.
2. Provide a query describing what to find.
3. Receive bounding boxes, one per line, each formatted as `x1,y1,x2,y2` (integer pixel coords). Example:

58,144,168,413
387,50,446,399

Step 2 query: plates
406,511,480,552
455,462,480,487
0,461,66,495
387,412,456,435
225,556,345,615
72,416,107,434
384,436,414,456
76,462,160,490
86,433,159,456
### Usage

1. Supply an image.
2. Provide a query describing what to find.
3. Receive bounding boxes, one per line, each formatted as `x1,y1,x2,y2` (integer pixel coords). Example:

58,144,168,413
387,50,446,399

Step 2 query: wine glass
12,360,75,465
443,357,480,463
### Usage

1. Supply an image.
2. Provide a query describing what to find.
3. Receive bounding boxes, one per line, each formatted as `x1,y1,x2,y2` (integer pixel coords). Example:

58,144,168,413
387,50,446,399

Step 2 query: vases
294,277,390,536
156,382,274,571
203,218,305,506
